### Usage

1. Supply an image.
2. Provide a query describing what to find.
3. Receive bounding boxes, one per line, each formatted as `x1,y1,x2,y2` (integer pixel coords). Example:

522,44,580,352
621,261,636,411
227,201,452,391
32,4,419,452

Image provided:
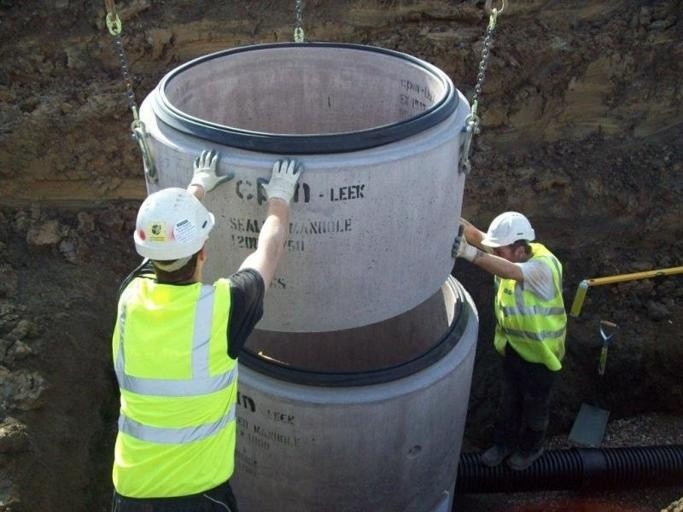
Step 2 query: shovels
568,320,620,448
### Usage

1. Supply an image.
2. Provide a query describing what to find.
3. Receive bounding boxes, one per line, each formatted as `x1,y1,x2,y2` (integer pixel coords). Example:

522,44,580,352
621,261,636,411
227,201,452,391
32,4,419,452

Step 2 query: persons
109,147,303,512
450,206,569,472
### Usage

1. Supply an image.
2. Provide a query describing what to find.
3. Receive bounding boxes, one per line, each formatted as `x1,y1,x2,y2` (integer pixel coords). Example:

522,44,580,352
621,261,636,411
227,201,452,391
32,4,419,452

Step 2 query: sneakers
506,448,544,471
480,445,513,467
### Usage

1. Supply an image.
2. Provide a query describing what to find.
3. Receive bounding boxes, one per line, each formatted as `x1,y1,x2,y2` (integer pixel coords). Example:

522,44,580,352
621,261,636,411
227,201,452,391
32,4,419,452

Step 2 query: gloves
190,148,234,193
261,159,303,204
450,224,477,263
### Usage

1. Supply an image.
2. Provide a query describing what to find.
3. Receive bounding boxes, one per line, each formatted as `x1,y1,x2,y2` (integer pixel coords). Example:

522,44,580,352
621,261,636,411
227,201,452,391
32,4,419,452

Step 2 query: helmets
479,211,536,249
132,187,215,261
